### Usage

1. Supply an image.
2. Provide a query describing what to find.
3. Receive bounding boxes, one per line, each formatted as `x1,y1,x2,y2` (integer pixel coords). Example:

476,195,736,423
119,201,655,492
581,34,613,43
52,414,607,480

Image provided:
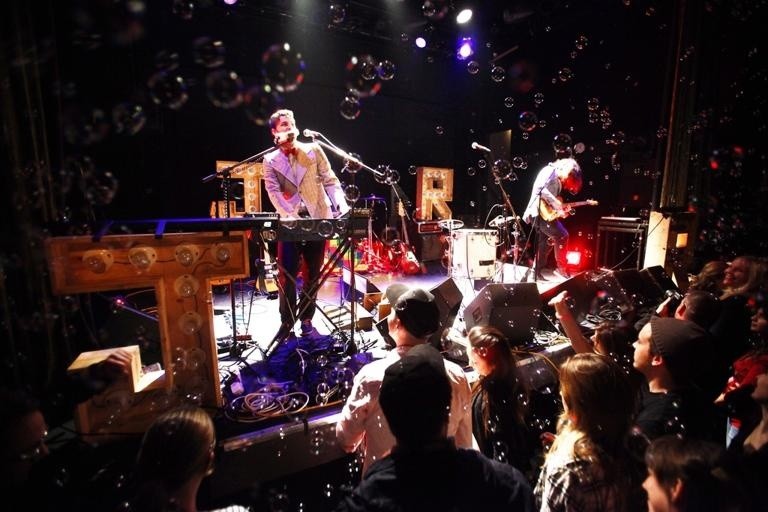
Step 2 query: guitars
395,199,420,274
255,221,279,292
539,196,599,221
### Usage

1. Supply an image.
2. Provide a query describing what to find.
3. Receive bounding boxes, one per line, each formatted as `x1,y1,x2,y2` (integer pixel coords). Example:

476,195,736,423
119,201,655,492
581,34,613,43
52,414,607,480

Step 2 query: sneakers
532,271,544,280
553,267,571,280
301,319,313,336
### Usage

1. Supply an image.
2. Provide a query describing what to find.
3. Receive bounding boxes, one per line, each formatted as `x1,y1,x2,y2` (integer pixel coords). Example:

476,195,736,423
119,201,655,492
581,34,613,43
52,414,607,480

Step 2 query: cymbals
439,219,464,231
490,216,520,228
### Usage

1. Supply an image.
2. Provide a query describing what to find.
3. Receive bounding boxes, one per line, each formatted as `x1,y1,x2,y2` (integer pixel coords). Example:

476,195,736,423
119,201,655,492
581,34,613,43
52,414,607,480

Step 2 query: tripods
201,173,262,382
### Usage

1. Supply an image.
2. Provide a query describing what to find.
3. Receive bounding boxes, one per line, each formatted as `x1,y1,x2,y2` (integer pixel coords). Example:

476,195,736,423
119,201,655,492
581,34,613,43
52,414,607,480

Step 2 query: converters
229,381,245,396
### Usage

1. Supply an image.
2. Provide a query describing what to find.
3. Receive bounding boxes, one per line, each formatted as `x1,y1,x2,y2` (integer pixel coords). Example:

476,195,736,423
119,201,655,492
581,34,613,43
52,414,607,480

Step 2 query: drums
449,229,497,278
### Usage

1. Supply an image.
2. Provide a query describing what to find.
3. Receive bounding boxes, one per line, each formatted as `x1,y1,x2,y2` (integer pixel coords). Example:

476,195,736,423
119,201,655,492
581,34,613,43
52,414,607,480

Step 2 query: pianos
162,217,349,244
599,216,647,236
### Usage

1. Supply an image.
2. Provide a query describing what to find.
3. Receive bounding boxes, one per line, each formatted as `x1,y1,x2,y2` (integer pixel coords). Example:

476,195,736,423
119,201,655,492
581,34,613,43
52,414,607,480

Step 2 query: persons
523,157,582,282
121,399,257,510
337,255,768,512
0,394,63,511
262,109,353,344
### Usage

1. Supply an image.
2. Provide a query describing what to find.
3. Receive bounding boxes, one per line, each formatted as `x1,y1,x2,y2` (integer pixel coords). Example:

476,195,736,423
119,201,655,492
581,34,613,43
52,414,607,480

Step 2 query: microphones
286,132,295,138
303,129,320,138
471,141,492,153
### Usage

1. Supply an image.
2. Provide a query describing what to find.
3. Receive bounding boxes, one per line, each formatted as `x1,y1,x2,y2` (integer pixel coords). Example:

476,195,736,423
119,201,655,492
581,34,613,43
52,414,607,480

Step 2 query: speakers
595,268,657,310
540,270,603,332
374,276,464,351
464,282,542,348
638,265,679,295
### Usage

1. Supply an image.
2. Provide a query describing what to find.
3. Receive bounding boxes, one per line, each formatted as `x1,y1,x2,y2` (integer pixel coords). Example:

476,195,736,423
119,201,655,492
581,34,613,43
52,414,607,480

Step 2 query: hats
378,343,445,427
387,283,441,337
651,315,706,378
